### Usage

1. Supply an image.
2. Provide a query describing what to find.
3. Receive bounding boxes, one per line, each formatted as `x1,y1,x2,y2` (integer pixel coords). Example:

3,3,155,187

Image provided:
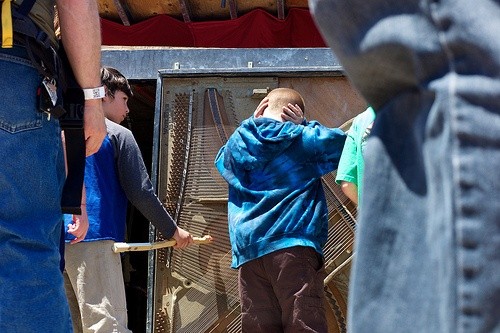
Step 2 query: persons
308,0,500,333
62,67,195,332
215,87,347,332
335,107,377,206
0,0,107,333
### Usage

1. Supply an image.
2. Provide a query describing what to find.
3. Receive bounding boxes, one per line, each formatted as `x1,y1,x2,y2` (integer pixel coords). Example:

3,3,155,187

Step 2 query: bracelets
81,203,86,206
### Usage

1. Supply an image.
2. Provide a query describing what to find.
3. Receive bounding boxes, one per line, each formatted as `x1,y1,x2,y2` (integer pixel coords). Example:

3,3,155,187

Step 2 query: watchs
81,87,107,99
